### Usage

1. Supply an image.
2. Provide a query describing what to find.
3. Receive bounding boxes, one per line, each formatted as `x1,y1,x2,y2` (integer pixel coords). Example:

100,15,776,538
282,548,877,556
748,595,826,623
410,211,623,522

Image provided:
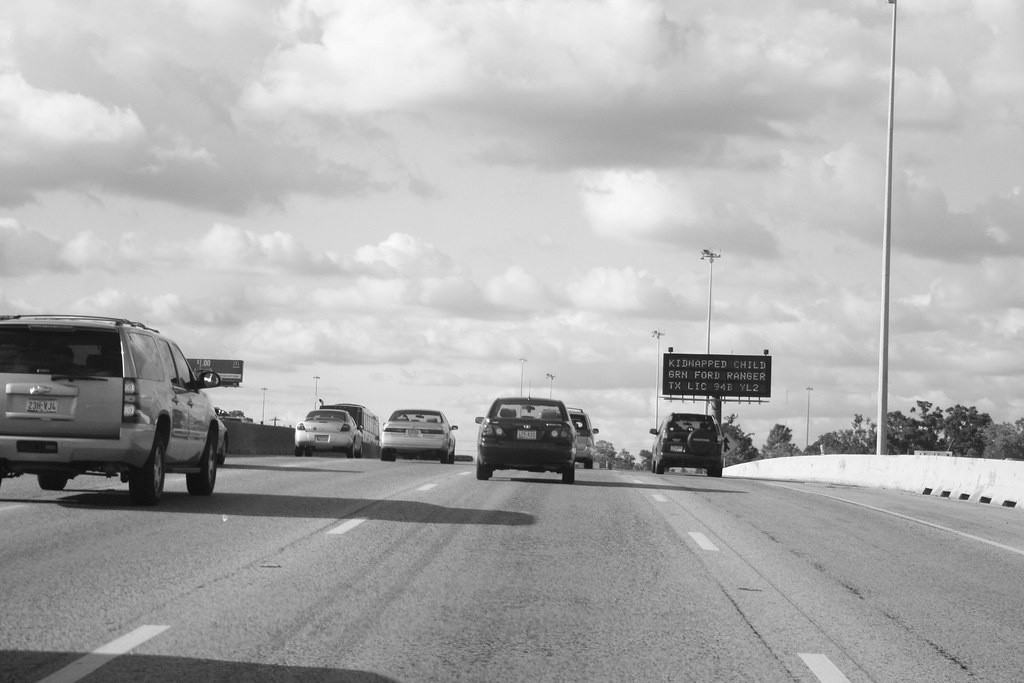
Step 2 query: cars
293,408,364,459
217,417,228,466
380,408,459,465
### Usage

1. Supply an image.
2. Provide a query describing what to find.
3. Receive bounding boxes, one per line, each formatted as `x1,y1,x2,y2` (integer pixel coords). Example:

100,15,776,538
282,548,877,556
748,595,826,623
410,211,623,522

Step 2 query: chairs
427,418,438,423
397,417,409,421
499,408,516,418
541,411,558,419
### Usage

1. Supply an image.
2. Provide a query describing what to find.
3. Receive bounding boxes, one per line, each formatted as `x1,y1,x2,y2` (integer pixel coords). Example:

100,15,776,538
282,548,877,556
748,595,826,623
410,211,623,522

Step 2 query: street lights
261,387,268,425
700,248,721,415
518,358,527,397
652,329,664,436
546,373,555,399
313,377,321,410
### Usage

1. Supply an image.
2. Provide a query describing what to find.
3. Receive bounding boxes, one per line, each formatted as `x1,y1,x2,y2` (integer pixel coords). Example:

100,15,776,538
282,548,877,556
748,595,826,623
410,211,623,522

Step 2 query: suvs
649,412,728,477
0,313,221,504
535,407,600,470
473,397,581,484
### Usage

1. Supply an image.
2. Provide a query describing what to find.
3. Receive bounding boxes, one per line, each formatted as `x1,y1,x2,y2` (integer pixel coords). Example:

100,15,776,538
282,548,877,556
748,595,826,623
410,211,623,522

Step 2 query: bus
318,398,381,445
